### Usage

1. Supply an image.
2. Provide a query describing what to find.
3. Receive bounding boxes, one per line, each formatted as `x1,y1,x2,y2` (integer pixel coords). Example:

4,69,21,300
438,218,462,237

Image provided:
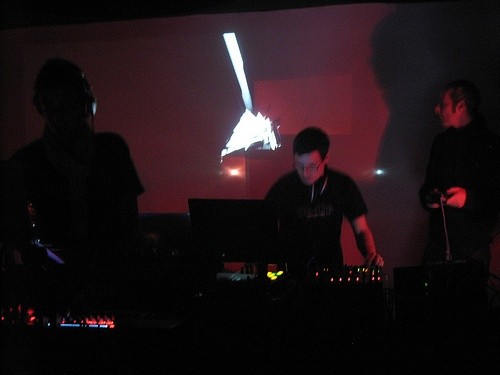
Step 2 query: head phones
32,58,96,119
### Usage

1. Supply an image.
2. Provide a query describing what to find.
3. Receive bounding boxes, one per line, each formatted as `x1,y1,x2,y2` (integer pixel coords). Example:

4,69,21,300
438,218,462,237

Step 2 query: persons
7,57,145,308
419,79,500,275
265,127,385,266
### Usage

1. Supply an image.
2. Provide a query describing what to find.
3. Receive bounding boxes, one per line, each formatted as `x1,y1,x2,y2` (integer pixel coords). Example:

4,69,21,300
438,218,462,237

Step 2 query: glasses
293,159,322,172
